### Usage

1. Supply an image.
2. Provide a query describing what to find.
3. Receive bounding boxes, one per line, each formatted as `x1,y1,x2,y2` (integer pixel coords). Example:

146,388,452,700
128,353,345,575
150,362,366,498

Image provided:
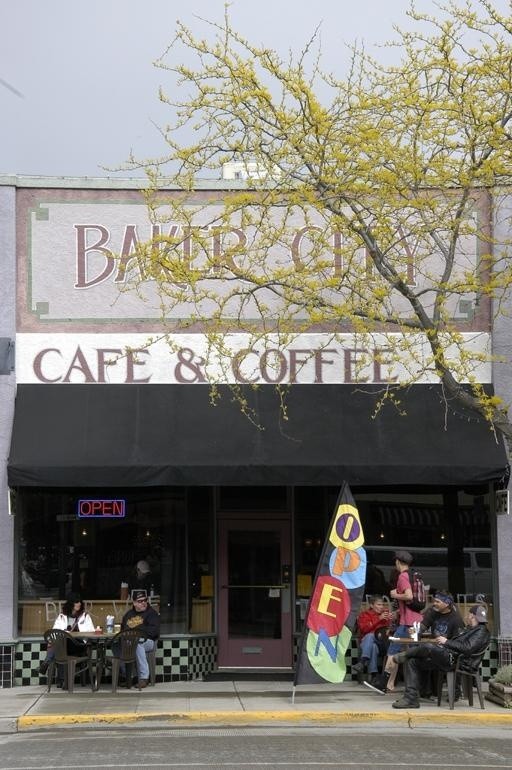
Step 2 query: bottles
37,545,74,561
149,584,155,596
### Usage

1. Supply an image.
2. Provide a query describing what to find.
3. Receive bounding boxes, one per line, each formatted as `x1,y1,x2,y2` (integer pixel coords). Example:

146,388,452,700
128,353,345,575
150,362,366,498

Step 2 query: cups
94,615,121,636
121,587,128,600
382,608,389,618
407,626,422,641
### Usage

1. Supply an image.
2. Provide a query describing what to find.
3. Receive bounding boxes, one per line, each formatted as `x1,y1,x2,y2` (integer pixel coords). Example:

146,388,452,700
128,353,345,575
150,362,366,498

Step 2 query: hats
393,550,413,564
133,591,147,601
136,560,153,575
469,605,489,624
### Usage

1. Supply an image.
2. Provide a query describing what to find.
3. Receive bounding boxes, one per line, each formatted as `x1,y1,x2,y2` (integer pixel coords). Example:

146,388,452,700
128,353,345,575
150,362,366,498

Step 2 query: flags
295,485,366,687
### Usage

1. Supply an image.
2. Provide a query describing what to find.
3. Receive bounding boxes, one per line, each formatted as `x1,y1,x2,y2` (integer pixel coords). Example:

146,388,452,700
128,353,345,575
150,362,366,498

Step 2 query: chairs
374,626,394,656
436,643,490,711
43,625,156,695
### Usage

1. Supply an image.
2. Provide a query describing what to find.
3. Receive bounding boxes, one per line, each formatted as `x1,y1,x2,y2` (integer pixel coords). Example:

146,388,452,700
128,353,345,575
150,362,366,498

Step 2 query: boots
35,662,49,674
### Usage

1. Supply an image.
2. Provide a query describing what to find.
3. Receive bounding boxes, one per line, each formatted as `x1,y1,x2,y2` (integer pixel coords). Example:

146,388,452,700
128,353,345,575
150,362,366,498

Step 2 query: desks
388,636,442,647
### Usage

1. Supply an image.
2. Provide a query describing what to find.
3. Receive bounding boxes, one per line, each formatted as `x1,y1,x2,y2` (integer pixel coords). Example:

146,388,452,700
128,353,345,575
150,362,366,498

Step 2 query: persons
363,550,425,696
390,603,491,709
118,588,160,691
418,587,460,642
120,558,158,600
32,590,95,689
353,589,392,680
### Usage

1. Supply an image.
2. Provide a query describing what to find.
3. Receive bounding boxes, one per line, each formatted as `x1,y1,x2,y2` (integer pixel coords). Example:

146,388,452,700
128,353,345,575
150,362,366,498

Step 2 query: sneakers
119,678,135,687
393,695,419,708
393,651,409,665
135,679,149,688
352,659,391,695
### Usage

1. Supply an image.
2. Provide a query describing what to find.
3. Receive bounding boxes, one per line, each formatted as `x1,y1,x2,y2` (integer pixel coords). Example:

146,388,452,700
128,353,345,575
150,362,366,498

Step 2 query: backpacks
396,567,426,611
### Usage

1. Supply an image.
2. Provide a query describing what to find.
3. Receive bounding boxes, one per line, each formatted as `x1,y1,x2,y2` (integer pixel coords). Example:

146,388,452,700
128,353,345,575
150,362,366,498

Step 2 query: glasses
74,600,82,603
138,598,147,603
